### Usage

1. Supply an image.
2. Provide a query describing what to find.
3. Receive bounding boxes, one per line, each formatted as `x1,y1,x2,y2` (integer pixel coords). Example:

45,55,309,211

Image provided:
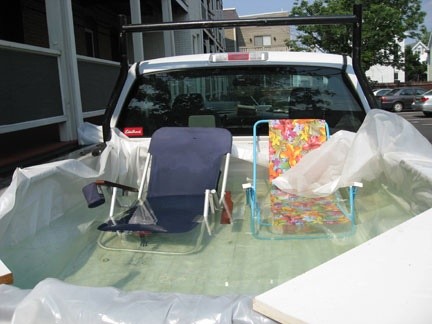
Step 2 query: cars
411,89,432,116
376,87,427,113
372,89,393,98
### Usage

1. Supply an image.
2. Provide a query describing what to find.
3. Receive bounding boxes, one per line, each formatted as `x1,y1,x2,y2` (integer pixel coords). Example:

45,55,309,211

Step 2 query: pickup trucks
0,3,432,324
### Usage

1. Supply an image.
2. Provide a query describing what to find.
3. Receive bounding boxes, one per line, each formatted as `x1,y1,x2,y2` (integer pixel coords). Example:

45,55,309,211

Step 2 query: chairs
242,118,363,240
171,87,326,127
96,126,234,255
